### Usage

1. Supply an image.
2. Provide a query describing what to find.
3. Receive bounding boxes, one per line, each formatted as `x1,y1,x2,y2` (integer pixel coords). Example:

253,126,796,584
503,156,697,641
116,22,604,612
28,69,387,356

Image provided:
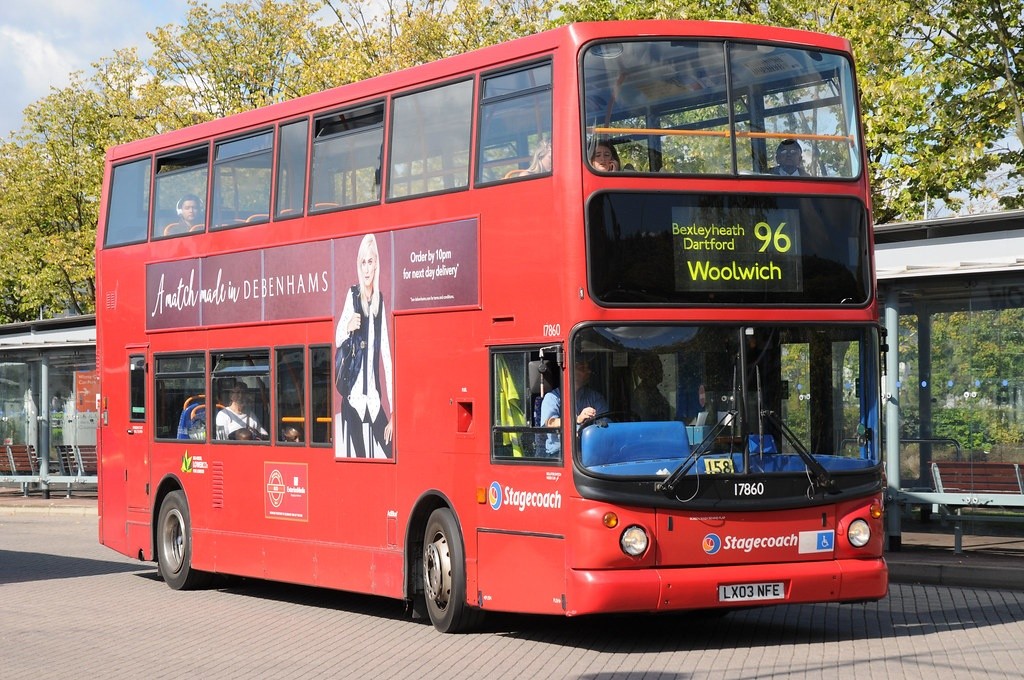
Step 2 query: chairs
162,203,343,237
535,397,559,460
132,377,227,440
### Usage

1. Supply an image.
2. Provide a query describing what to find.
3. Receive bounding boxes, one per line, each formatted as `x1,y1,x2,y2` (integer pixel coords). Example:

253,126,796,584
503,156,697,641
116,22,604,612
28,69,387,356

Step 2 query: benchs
926,461,1024,556
56,445,96,500
0,445,39,497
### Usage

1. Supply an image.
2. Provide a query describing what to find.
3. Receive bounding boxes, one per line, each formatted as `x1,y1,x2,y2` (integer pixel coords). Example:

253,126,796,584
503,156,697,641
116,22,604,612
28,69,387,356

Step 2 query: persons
214,383,269,442
629,352,674,422
769,140,809,176
169,194,202,236
285,426,303,442
541,355,609,459
518,140,550,176
334,232,392,458
236,428,253,440
588,138,622,173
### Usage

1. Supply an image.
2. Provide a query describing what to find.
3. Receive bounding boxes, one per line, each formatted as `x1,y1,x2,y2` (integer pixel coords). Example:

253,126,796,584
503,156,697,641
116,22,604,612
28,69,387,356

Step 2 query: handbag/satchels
335,286,365,398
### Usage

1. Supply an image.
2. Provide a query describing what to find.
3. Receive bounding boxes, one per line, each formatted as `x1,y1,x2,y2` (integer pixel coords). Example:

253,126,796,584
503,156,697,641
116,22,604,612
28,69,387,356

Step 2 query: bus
94,19,891,635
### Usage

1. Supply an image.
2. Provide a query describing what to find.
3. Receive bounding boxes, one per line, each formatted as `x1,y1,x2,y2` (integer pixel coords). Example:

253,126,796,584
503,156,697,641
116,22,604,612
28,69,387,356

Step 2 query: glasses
780,149,798,155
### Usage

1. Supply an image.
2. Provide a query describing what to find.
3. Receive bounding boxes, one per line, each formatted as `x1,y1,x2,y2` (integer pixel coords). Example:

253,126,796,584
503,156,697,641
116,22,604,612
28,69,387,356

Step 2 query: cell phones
609,155,615,171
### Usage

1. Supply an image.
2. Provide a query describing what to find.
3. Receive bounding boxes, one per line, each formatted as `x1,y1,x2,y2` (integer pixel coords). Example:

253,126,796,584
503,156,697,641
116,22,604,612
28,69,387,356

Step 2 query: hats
629,349,660,376
574,353,594,367
